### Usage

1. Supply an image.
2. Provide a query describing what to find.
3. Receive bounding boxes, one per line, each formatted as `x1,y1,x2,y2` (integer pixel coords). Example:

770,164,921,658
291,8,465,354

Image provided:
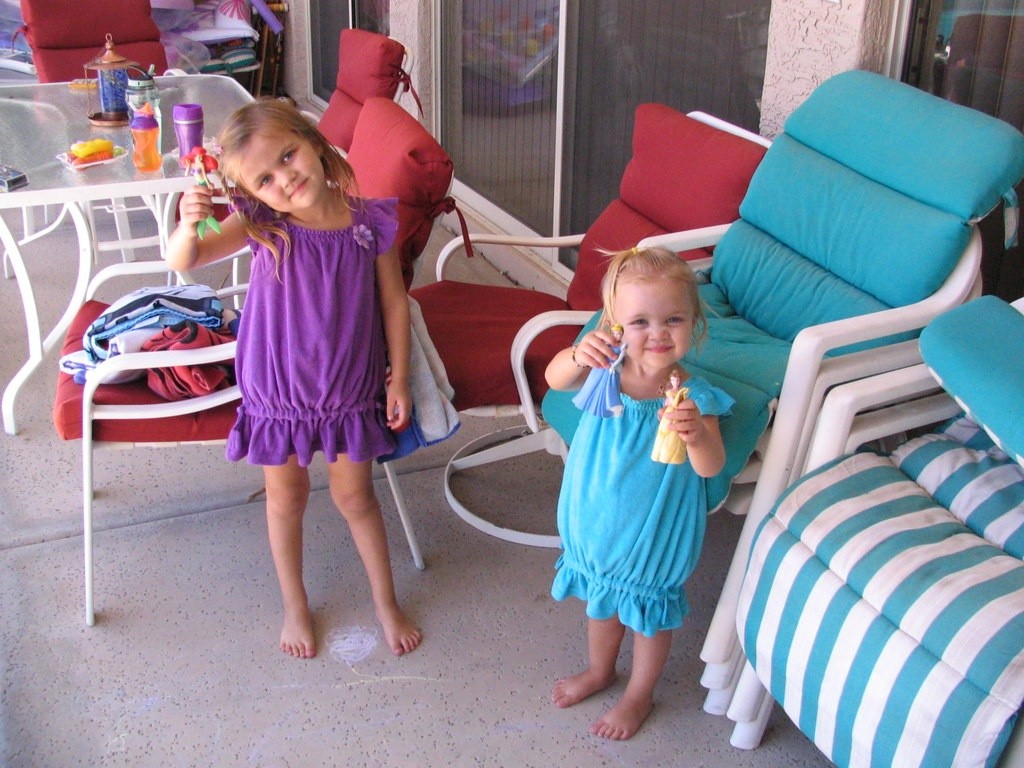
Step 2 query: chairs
163,27,425,287
51,94,475,628
11,0,168,226
410,99,774,549
543,68,1024,768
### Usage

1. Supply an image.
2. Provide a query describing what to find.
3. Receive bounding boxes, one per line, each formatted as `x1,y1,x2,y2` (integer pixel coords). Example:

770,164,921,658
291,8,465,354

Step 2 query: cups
173,104,205,161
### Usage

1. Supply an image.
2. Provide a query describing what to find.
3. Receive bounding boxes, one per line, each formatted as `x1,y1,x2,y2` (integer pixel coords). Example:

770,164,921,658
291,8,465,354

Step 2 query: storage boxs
55,144,129,176
0,165,30,192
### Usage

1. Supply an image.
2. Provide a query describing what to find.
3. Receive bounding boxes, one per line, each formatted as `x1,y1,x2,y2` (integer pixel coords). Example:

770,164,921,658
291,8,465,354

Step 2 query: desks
0,72,257,439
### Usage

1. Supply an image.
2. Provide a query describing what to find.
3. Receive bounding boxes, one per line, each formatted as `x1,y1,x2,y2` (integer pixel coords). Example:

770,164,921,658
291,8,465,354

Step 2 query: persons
166,100,423,657
543,247,738,742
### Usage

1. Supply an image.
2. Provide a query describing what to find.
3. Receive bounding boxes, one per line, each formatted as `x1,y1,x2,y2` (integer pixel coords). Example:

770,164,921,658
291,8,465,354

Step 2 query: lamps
84,33,143,128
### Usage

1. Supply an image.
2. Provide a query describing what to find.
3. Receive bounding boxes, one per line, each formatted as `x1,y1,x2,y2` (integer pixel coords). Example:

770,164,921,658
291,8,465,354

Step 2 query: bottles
124,65,162,155
129,102,162,173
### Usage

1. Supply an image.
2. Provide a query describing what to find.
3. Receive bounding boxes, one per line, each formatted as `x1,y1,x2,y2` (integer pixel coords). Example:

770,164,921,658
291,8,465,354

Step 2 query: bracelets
572,343,590,369
